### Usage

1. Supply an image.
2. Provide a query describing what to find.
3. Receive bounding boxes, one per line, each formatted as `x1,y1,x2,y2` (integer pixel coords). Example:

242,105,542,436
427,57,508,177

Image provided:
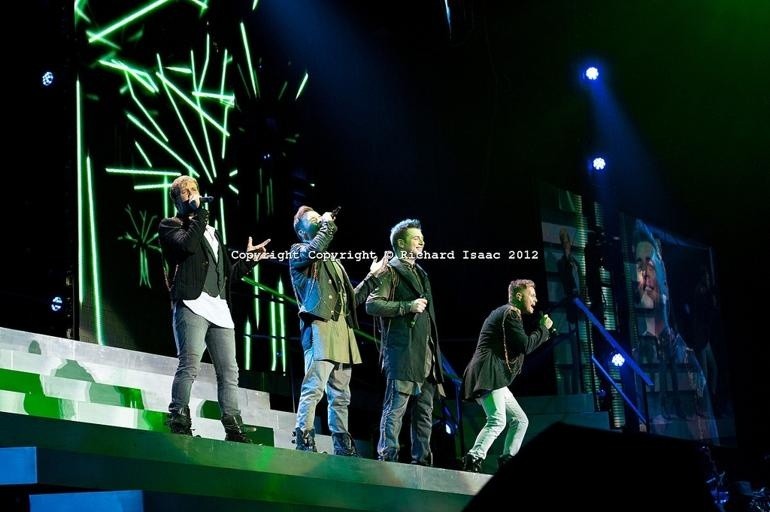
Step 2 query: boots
291,427,327,454
332,431,359,457
168,403,191,437
221,414,255,443
461,454,483,474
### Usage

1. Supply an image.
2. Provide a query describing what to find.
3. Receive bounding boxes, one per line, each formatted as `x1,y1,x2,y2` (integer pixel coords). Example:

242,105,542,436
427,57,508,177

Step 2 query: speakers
463,420,721,512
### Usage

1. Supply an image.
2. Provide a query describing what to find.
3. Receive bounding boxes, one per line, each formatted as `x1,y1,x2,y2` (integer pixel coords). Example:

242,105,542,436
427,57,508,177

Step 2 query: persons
627,217,722,440
557,226,588,393
159,166,273,445
365,217,449,468
286,201,390,458
458,279,555,472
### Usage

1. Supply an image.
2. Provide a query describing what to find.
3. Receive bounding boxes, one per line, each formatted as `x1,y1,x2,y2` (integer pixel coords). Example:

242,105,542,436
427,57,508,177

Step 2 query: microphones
537,310,560,338
331,206,342,219
409,292,427,328
199,196,214,203
